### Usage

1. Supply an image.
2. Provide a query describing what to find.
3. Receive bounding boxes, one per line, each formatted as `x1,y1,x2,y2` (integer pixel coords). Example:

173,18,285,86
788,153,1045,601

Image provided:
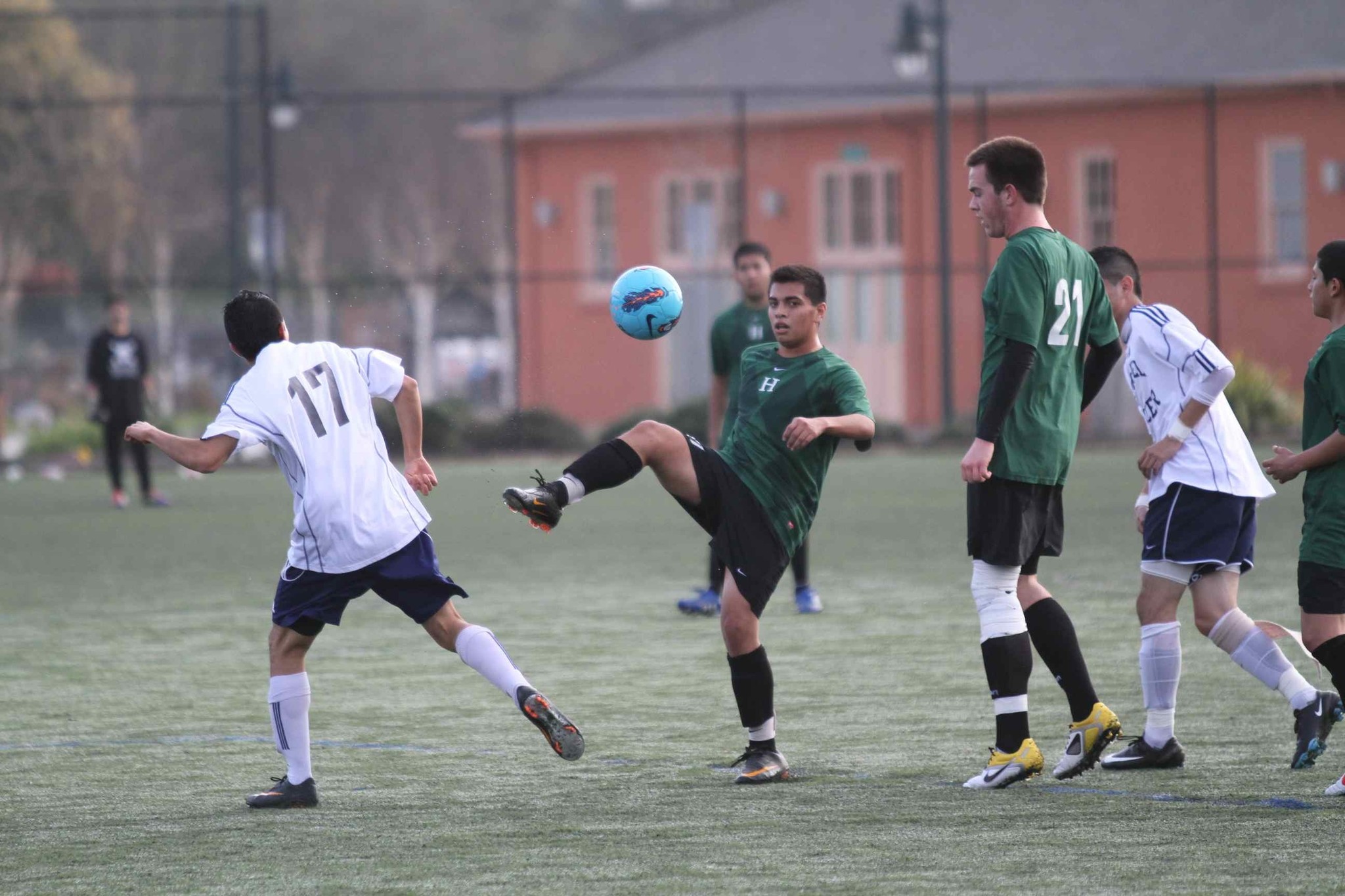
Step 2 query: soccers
609,265,684,340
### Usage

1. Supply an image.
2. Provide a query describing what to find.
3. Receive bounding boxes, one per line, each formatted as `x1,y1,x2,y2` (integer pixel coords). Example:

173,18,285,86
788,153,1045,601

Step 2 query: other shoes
144,494,168,506
111,492,128,508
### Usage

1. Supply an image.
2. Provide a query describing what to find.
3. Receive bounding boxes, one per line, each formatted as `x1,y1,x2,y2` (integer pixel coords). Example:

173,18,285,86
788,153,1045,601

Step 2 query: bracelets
1167,418,1191,443
1135,493,1150,510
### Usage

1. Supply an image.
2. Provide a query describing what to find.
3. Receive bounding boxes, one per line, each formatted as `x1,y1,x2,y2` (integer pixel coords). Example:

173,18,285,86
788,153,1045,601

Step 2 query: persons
500,265,878,787
125,290,585,808
676,242,822,615
1090,248,1345,772
960,135,1124,791
85,293,172,511
1260,238,1345,797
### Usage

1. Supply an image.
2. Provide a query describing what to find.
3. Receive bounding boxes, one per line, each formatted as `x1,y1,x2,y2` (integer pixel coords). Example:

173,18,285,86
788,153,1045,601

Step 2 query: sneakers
502,486,562,532
1324,770,1345,795
677,589,722,615
1290,690,1343,769
962,738,1044,789
734,748,790,784
1102,734,1186,769
516,686,585,760
794,586,823,613
245,776,318,807
1052,702,1123,779
